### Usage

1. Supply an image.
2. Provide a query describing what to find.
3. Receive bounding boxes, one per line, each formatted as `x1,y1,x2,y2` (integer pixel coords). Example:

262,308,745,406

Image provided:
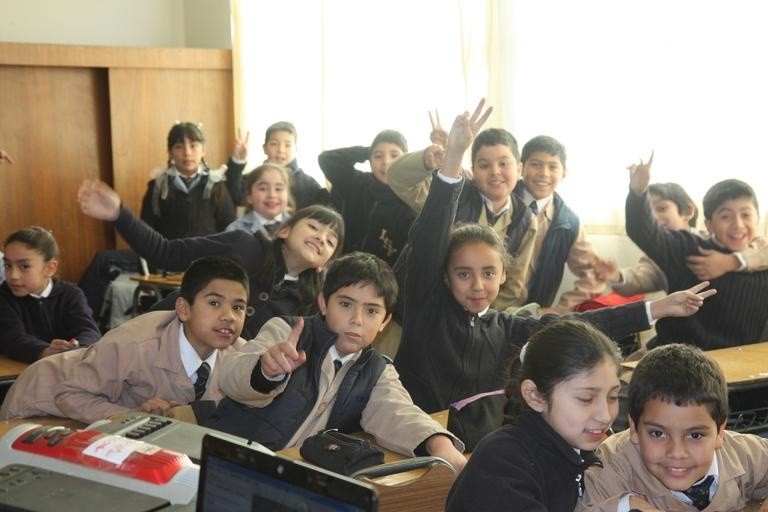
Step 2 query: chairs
350,456,457,512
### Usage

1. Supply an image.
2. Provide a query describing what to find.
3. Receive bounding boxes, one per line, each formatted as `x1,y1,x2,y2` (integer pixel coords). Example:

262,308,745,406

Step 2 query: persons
75,120,346,343
586,180,767,299
0,253,250,427
393,95,717,414
0,224,100,364
442,311,621,511
206,251,468,474
317,105,607,361
575,343,767,511
618,147,768,353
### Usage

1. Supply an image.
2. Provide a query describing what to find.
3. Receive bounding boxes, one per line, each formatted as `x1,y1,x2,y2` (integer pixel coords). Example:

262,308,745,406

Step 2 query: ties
674,475,714,511
528,201,537,215
483,198,508,227
194,362,211,400
